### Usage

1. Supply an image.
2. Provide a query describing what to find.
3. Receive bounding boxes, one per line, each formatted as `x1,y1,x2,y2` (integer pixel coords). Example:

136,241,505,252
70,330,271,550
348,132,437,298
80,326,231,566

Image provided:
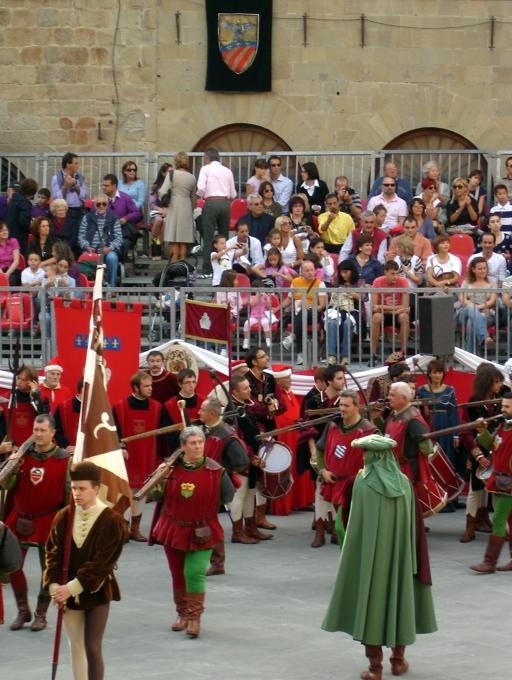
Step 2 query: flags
73,274,131,503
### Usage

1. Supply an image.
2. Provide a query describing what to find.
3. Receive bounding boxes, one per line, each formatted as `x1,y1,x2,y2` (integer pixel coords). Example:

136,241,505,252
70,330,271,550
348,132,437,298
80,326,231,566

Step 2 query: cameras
346,186,355,195
297,225,308,233
398,255,412,267
237,244,243,249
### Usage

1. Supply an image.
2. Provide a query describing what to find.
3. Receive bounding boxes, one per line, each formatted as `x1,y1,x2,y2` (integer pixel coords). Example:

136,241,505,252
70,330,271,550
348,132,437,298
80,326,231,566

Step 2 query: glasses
95,201,108,209
281,221,292,226
428,187,437,189
265,189,274,193
252,202,263,206
125,169,136,172
257,353,266,360
413,204,424,209
271,163,281,167
453,185,463,189
382,184,396,187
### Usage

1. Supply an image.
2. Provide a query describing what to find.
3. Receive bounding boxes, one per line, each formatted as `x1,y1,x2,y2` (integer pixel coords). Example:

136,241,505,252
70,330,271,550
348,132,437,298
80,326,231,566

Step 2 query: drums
427,442,466,502
475,452,495,482
399,459,448,520
257,440,296,501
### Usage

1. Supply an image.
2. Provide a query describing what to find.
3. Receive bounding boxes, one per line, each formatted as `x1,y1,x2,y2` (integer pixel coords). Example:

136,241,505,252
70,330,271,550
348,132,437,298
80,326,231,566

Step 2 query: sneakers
209,272,214,279
368,356,378,367
328,357,336,366
264,338,274,347
242,339,249,350
282,339,289,350
197,272,208,279
296,361,306,365
340,356,348,366
487,339,497,348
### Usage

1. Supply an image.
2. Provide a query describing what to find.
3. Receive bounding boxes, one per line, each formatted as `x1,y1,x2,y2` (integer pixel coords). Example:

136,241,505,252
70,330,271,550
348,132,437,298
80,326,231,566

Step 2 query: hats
68,459,101,482
383,351,405,365
270,363,292,378
7,180,21,190
351,432,409,499
231,359,250,371
45,355,63,371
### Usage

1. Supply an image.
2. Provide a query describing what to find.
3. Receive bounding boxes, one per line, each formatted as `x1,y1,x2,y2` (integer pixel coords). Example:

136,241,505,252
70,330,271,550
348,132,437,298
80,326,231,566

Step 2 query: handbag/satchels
330,289,355,310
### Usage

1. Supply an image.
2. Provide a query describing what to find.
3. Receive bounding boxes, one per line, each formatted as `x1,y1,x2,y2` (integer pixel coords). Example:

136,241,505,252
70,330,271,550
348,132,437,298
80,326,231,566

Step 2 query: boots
362,644,385,680
229,520,259,544
170,589,187,631
126,514,150,542
496,539,512,572
470,535,507,574
185,594,205,637
31,592,52,631
389,646,410,674
10,590,31,631
477,508,494,533
311,517,326,548
330,519,342,546
243,517,273,540
255,503,276,529
459,513,476,543
205,540,228,575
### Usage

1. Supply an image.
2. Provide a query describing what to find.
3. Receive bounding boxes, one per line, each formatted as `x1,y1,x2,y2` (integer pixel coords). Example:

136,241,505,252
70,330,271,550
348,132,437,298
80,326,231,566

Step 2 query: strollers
146,251,199,344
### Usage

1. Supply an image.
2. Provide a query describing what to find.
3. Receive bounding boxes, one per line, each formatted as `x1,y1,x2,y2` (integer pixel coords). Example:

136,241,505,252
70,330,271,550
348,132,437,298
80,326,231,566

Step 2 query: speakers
417,295,454,356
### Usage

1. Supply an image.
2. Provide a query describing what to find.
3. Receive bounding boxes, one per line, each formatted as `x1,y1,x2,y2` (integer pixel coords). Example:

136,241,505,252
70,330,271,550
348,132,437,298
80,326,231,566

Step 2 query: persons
195,147,237,278
1,151,196,339
39,355,83,445
42,463,125,644
114,350,218,540
209,223,264,339
0,356,71,632
